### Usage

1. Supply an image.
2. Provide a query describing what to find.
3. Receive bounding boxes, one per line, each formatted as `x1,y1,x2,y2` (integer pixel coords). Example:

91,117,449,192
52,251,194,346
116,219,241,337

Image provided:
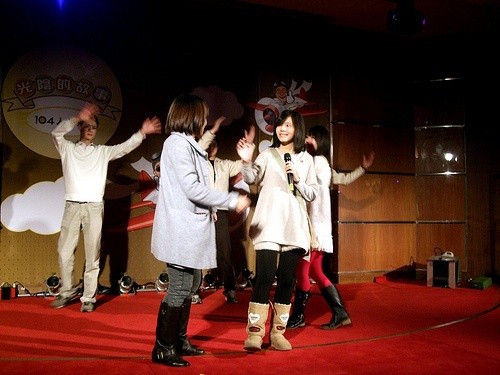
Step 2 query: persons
191,117,255,304
331,150,375,185
287,126,351,329
236,110,318,350
152,95,252,367
50,103,162,312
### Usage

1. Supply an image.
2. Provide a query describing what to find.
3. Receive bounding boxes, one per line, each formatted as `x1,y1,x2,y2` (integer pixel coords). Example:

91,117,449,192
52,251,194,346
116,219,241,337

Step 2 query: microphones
284,153,294,190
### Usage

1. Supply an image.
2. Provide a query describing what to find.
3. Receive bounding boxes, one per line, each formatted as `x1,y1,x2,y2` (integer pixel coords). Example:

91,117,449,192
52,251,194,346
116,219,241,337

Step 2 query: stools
426,256,461,288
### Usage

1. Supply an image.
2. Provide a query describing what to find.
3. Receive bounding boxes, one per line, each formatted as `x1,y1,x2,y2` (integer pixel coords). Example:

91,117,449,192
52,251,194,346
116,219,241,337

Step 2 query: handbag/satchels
306,213,316,250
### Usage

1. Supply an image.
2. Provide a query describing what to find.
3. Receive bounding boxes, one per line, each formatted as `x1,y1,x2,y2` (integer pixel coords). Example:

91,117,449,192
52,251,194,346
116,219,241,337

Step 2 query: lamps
236,268,251,287
155,272,171,292
118,275,133,294
203,271,217,288
45,271,62,296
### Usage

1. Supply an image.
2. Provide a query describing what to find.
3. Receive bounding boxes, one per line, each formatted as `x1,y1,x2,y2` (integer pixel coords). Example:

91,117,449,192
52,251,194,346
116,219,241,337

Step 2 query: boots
285,288,312,329
152,302,189,367
270,302,293,351
243,301,270,352
178,298,205,356
320,284,351,329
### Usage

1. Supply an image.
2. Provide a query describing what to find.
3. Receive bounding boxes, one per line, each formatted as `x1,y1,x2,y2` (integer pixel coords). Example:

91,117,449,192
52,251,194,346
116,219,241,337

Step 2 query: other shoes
50,291,78,309
80,302,94,312
190,292,202,304
225,289,238,303
